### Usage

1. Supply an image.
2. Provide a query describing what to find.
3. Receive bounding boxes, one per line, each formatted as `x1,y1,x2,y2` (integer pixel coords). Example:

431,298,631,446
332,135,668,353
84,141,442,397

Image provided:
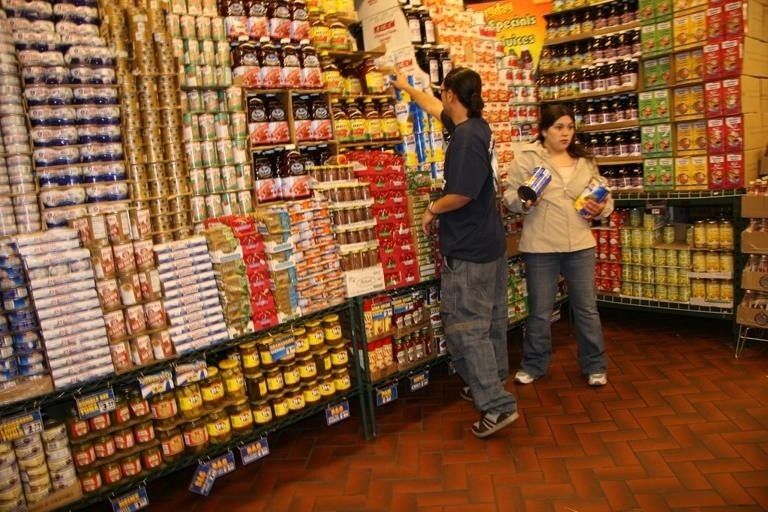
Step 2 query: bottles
572,41,584,67
342,185,352,201
656,285,665,300
121,453,142,478
690,278,706,302
66,405,89,437
365,225,374,241
614,129,628,155
251,401,274,425
324,315,342,342
600,245,609,260
610,230,620,245
538,71,580,100
641,249,653,266
361,52,386,94
270,1,291,39
601,131,615,156
309,93,334,140
641,231,651,248
306,319,324,349
594,263,600,278
620,280,632,296
693,219,706,247
291,91,312,144
378,97,402,139
232,36,261,87
581,9,594,32
158,420,186,464
330,97,350,140
558,14,569,38
593,247,599,259
345,97,368,140
142,448,163,473
247,376,268,405
224,0,248,39
620,265,631,280
353,205,363,222
333,346,349,369
339,251,351,271
357,225,365,242
291,1,312,42
101,457,123,487
403,4,422,45
439,48,453,83
706,253,719,274
592,36,605,64
721,219,733,248
604,32,617,62
591,229,599,246
686,224,696,247
368,246,378,266
184,416,210,459
610,264,621,279
720,251,734,274
364,97,384,140
718,280,734,303
331,185,343,202
92,415,112,435
680,287,690,302
595,4,607,28
152,386,176,424
266,93,290,144
71,434,93,467
611,280,620,294
607,62,620,88
706,278,716,303
297,355,317,382
300,38,322,89
254,149,277,202
419,7,434,45
363,204,373,221
580,66,593,94
80,462,104,496
598,98,612,123
281,145,309,199
644,262,653,284
394,286,424,330
220,358,244,395
336,166,345,180
288,386,306,412
347,227,358,243
610,246,620,262
600,264,611,279
655,247,665,266
574,176,609,220
259,36,282,89
617,163,630,187
630,249,643,265
95,433,116,463
611,95,621,121
562,42,572,69
131,388,149,418
404,336,416,364
411,332,426,360
230,397,254,433
594,63,608,91
279,37,303,89
631,163,644,188
342,57,362,94
633,283,643,298
239,341,258,374
609,208,621,229
665,250,677,267
629,205,640,226
318,375,337,399
668,269,678,286
318,51,343,92
545,17,558,40
310,166,321,182
335,207,344,225
116,424,136,454
421,329,433,356
583,98,598,125
631,58,640,88
584,41,593,65
336,367,351,390
601,280,609,292
616,32,632,60
275,332,294,363
283,360,301,388
632,27,643,57
247,92,270,144
350,183,361,200
315,349,331,375
267,366,283,395
312,19,330,50
111,395,133,427
272,392,289,418
622,246,632,263
602,165,617,188
419,44,439,84
620,0,633,24
643,285,655,300
177,383,205,419
300,145,316,177
393,71,450,182
708,218,720,249
335,229,348,244
359,182,370,199
137,424,158,448
518,166,551,203
320,165,325,181
653,250,665,266
247,1,270,39
393,337,407,367
326,165,336,180
619,229,629,245
259,337,275,368
599,230,609,246
620,95,628,111
552,46,562,69
208,410,232,448
569,10,581,35
351,249,361,269
625,93,638,119
692,252,706,272
668,285,679,299
634,266,642,284
290,327,310,357
607,1,620,26
594,279,601,292
632,230,641,247
586,132,601,156
656,266,666,281
301,381,321,405
330,23,347,51
662,223,674,245
344,206,354,224
679,248,693,269
306,167,310,176
345,165,353,181
360,247,369,268
622,59,635,87
629,129,641,156
678,269,689,284
539,46,554,70
201,366,224,408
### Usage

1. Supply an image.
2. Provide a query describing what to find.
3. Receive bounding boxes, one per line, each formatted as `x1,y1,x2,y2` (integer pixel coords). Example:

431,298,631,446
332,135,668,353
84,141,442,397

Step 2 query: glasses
435,87,450,94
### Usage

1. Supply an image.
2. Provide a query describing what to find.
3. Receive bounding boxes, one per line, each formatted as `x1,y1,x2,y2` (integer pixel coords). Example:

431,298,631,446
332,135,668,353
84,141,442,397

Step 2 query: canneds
0,421,75,510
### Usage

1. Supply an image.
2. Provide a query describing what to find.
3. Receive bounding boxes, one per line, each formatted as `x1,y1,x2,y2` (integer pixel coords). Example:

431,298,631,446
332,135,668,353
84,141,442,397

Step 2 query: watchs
428,200,436,216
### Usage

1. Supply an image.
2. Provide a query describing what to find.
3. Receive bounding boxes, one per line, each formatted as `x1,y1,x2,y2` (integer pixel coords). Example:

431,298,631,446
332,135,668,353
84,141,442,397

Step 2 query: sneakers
513,369,538,386
585,369,608,387
456,385,480,403
467,404,520,439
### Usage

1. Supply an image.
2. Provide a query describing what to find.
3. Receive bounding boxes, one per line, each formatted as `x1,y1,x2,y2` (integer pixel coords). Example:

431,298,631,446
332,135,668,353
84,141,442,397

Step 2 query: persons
503,105,615,386
391,67,520,438
